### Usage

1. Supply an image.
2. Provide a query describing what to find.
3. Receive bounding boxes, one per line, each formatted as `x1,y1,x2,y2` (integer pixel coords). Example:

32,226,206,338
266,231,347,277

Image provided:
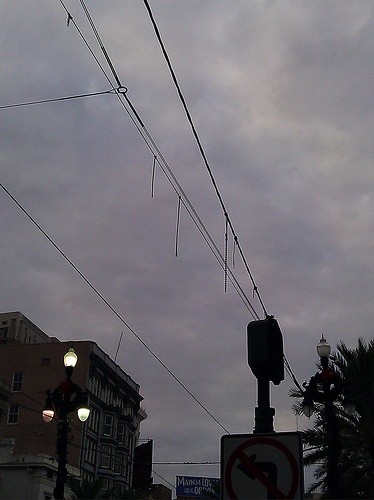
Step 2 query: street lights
42,348,90,500
